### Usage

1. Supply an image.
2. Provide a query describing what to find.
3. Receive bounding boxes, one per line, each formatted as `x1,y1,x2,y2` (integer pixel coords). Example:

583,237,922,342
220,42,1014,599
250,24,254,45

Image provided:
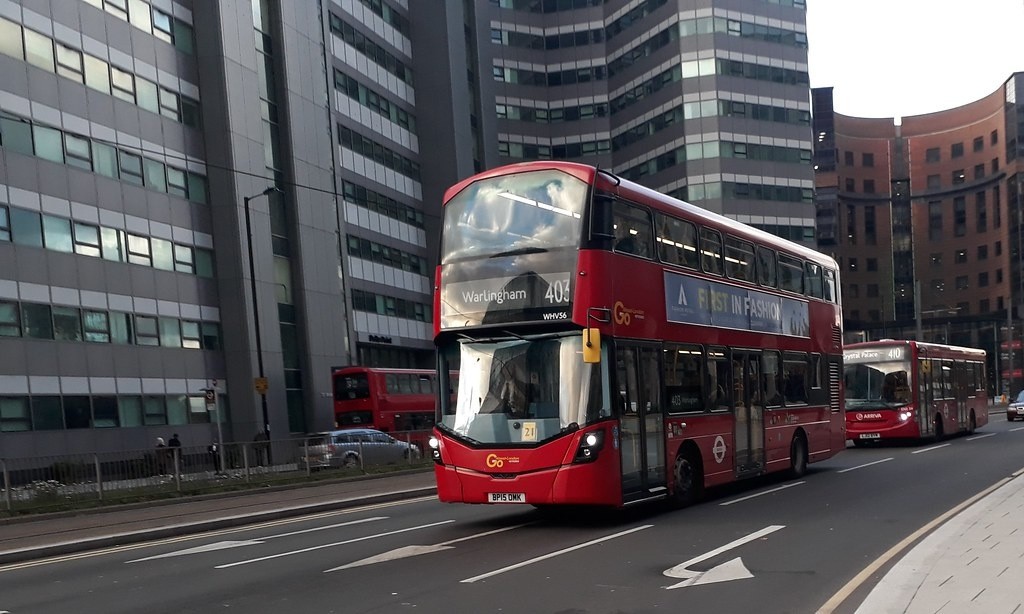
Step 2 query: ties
503,383,511,411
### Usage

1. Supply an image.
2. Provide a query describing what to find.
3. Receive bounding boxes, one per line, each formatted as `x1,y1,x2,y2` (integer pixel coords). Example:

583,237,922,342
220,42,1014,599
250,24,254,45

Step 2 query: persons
493,363,530,419
208,437,222,475
155,434,183,478
254,428,269,467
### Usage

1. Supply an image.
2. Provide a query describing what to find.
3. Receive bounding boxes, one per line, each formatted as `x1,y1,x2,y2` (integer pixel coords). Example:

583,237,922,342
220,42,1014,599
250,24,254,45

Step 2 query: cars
296,428,421,474
1006,390,1024,422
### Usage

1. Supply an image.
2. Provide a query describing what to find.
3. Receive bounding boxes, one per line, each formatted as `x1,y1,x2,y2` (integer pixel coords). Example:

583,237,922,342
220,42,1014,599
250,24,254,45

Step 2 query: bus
429,159,845,512
332,365,461,459
843,339,990,446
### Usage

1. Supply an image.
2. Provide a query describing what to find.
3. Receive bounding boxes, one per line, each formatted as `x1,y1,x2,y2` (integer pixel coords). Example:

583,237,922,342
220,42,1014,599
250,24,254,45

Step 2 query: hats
155,437,165,447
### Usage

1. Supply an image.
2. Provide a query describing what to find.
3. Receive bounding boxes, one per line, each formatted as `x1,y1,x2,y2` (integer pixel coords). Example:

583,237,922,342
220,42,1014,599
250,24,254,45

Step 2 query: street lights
244,185,279,468
